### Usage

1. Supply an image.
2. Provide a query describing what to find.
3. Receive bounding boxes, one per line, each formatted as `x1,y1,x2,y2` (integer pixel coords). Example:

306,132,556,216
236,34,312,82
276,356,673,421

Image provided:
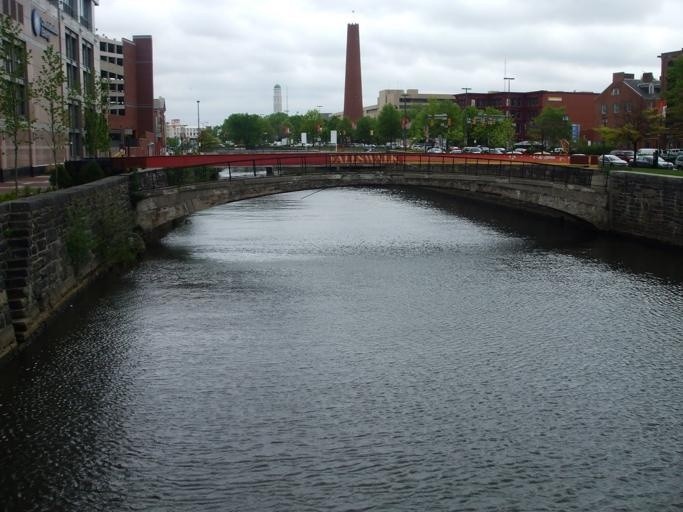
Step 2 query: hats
220,139,326,148
347,140,563,156
597,146,682,169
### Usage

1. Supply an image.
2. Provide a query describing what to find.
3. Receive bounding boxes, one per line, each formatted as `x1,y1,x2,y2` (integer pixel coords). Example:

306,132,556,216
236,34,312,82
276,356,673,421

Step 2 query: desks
562,113,569,154
503,77,515,122
195,99,200,137
460,87,471,107
399,93,411,152
